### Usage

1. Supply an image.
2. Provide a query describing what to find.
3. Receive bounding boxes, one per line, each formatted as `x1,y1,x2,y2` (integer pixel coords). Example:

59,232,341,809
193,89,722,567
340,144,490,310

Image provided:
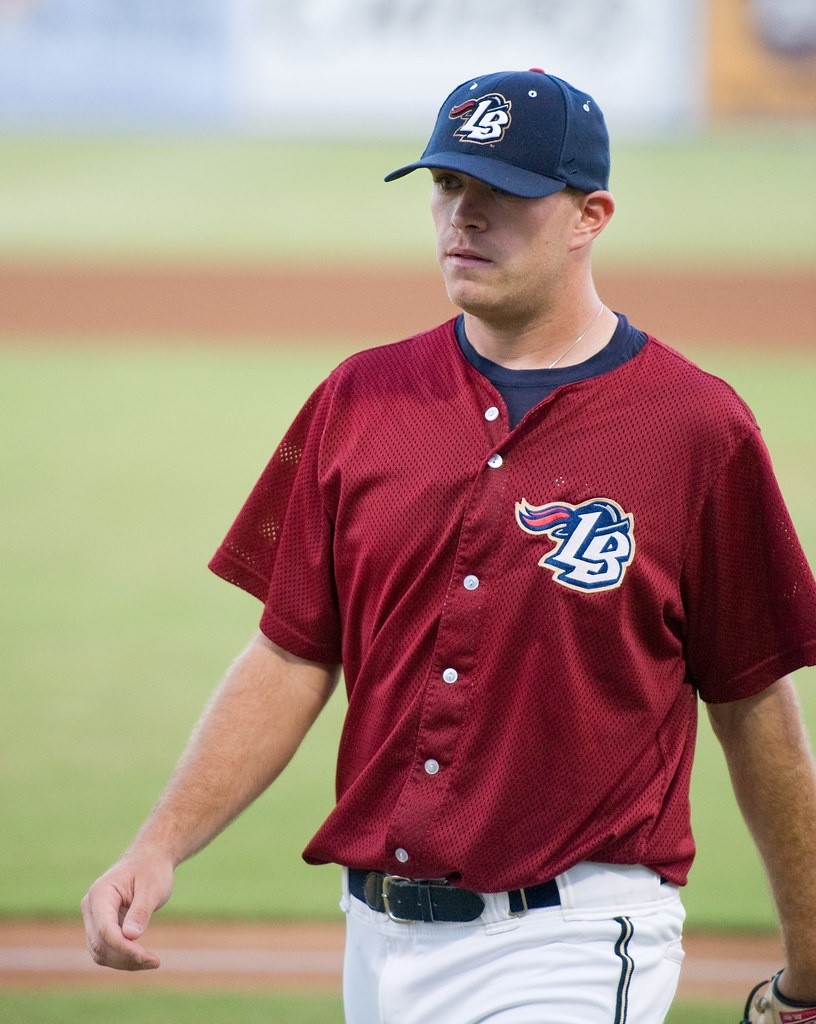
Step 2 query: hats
384,67,610,199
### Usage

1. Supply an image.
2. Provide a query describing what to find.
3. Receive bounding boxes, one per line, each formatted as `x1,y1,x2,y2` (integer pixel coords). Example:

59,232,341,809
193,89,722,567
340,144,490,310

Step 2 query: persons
80,74,816,1023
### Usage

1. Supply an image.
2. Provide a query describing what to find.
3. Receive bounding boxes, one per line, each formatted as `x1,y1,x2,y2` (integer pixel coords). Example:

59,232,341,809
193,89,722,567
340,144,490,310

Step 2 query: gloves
752,964,815,1024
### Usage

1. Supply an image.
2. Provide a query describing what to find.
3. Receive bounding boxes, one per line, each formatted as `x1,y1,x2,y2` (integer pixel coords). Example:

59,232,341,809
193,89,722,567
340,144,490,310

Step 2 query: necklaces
548,303,603,369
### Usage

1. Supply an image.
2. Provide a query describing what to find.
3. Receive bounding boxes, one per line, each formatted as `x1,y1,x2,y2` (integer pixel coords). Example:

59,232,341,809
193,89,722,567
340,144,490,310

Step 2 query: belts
347,866,670,925
